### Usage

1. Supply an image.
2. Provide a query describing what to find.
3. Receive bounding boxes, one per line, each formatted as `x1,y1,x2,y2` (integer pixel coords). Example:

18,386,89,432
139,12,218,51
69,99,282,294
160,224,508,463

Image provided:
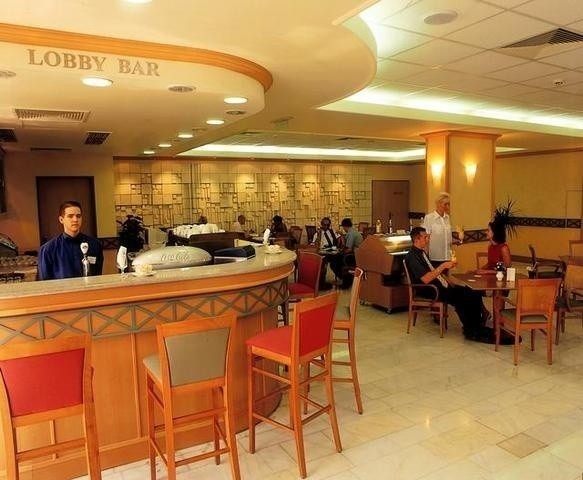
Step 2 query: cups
172,223,226,240
330,244,337,250
527,268,537,279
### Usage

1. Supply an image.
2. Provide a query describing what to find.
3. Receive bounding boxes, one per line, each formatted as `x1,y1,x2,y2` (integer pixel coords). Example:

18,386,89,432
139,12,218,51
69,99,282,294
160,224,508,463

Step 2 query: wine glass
457,229,465,244
115,253,129,278
448,250,459,271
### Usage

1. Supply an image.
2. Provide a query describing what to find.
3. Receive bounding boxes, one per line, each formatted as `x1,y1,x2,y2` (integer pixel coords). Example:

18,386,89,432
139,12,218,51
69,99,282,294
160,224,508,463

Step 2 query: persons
271,215,287,234
37,201,104,280
421,191,454,325
311,216,343,250
406,227,484,340
230,214,249,238
479,221,512,326
330,218,362,290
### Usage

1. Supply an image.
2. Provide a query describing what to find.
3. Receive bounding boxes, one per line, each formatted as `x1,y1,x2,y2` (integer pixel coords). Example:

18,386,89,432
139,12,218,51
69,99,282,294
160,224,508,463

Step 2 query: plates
264,250,283,255
131,272,158,278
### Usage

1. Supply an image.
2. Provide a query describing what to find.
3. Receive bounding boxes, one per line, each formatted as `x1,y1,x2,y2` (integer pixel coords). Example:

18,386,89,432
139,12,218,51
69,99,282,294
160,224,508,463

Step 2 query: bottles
375,218,417,235
82,255,91,276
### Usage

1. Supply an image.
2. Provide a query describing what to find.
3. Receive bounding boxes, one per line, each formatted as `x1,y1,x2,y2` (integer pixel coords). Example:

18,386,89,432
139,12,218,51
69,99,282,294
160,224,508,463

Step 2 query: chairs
1,329,102,480
402,244,581,367
237,220,376,289
242,288,343,480
139,309,246,480
298,266,367,416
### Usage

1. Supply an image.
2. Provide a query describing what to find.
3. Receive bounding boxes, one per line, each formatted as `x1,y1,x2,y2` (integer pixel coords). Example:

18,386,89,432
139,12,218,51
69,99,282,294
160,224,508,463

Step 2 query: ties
324,231,332,245
421,251,449,288
